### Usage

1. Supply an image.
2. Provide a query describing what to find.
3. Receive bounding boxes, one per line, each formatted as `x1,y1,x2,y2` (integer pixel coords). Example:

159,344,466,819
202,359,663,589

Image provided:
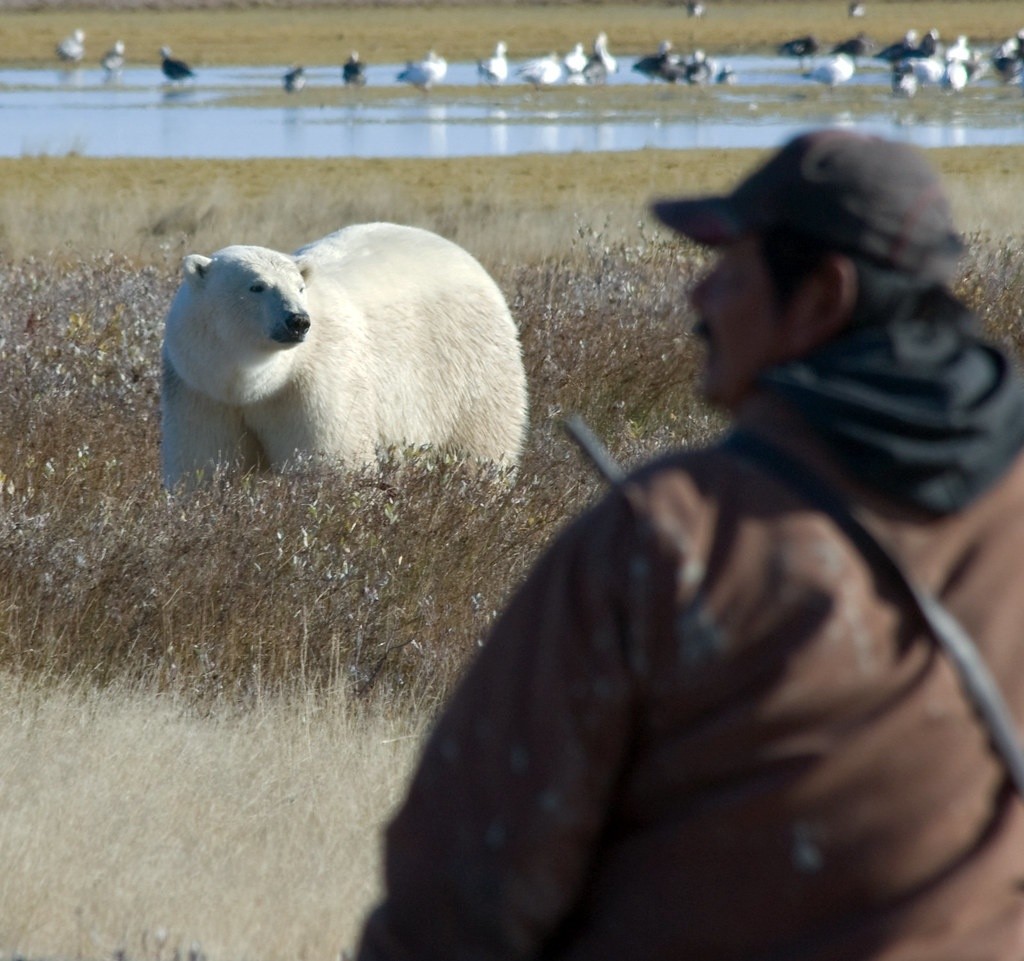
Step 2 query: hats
647,129,956,283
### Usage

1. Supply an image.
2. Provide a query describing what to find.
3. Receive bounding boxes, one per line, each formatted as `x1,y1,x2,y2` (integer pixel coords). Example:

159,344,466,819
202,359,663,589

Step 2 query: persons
354,127,1023,961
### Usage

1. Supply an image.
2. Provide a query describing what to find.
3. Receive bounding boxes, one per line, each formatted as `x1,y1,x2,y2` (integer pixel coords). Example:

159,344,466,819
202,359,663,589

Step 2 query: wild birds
53,0,1024,102
